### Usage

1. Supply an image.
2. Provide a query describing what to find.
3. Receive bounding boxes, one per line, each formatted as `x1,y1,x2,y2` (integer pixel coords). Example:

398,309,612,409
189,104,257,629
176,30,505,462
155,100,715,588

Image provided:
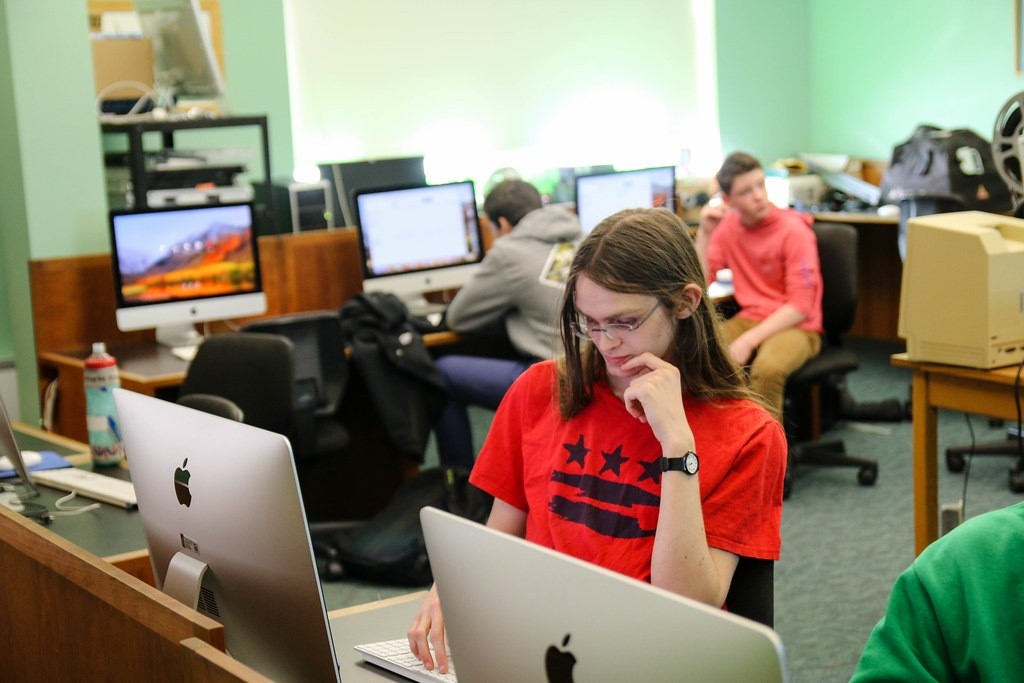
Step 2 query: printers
898,210,1024,370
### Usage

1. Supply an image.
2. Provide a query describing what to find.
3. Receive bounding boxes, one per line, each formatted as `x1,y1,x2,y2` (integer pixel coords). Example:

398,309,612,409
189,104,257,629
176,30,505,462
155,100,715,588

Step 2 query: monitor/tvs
351,179,484,318
112,387,342,683
109,201,267,348
0,397,30,515
574,165,677,236
133,1,226,121
419,505,789,683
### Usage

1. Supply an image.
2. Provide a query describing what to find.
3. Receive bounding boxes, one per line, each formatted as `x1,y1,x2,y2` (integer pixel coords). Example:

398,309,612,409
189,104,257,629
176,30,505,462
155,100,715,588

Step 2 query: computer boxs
254,180,335,235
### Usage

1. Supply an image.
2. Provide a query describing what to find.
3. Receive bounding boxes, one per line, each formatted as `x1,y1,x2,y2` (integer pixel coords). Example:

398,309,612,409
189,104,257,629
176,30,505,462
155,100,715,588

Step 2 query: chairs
1,229,866,683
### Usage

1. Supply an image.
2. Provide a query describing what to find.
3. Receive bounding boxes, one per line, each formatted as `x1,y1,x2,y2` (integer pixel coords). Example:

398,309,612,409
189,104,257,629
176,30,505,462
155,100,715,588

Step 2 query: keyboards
353,638,457,683
172,347,197,360
30,467,139,509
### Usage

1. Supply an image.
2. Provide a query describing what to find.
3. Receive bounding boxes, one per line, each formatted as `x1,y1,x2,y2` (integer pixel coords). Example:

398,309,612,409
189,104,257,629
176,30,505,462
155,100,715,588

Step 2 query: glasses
569,298,665,341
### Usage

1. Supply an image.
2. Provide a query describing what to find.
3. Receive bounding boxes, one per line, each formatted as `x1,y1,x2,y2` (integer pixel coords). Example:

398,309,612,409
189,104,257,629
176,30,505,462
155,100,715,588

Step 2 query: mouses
0,451,41,472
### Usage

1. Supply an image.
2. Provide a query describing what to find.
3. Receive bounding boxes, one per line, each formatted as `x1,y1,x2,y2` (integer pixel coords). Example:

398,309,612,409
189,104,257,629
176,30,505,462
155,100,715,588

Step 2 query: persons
407,207,787,674
425,180,592,503
695,152,822,423
848,500,1024,683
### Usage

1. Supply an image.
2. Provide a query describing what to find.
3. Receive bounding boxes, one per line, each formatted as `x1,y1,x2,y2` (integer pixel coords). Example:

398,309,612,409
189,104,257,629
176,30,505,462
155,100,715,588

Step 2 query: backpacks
339,466,473,585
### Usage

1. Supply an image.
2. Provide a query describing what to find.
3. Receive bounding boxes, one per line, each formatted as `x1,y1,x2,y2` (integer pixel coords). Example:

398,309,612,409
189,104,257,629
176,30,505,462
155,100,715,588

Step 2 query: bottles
82,343,124,466
707,269,734,299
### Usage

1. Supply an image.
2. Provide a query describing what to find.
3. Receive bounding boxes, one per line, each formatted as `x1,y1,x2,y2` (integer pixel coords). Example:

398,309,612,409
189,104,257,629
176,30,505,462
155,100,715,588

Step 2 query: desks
889,350,1023,562
0,421,430,683
36,297,498,443
97,112,272,213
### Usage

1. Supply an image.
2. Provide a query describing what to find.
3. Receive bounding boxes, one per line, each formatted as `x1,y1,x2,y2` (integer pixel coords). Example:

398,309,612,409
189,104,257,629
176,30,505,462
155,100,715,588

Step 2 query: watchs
658,452,700,475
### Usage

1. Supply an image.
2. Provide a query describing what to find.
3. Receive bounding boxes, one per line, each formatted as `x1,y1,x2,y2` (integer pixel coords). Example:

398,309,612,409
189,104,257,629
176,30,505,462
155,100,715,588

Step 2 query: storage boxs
86,33,154,113
